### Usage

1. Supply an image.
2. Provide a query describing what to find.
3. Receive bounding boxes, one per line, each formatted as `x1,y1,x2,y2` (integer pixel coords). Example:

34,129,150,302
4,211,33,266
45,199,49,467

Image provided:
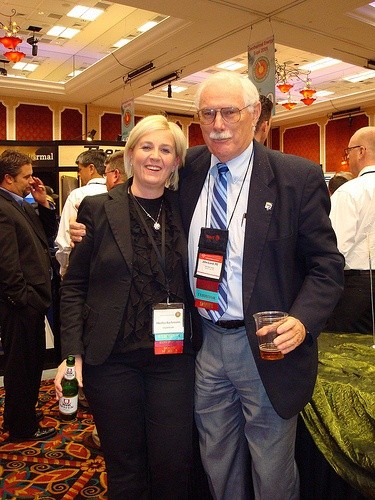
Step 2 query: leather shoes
9,409,57,441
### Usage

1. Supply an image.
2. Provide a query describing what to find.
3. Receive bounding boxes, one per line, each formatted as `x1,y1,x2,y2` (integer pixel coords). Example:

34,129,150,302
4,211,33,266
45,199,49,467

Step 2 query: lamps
0,8,26,76
275,58,316,111
72,128,97,141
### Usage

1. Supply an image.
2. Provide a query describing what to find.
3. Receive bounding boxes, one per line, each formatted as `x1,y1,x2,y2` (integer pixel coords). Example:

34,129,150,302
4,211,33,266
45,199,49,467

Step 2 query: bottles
58,354,78,421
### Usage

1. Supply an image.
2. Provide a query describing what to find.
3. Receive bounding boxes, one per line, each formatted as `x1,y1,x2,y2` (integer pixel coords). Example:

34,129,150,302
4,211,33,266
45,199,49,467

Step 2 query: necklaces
129,185,164,232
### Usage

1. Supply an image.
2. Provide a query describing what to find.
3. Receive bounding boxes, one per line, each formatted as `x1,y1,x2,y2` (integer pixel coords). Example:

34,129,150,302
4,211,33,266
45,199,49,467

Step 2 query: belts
203,317,245,329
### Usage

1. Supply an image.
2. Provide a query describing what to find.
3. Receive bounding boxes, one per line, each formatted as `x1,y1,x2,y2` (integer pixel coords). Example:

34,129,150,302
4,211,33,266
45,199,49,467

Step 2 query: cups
253,311,287,360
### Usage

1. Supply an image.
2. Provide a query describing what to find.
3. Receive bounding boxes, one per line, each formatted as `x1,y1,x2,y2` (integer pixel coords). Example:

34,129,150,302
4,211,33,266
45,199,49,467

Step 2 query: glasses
196,104,254,125
343,146,361,157
102,169,116,179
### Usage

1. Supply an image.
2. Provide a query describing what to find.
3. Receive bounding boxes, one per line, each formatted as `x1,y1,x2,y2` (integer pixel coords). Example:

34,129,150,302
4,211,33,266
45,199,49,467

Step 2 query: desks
297,332,375,500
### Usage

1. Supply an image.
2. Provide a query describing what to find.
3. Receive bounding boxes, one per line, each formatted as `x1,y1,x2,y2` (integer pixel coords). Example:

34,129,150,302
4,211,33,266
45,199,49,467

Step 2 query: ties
204,162,230,324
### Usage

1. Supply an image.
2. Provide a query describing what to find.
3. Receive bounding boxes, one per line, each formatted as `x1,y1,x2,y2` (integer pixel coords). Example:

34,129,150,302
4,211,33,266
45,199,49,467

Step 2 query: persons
103,152,127,194
328,171,357,200
54,150,109,284
328,125,375,338
0,149,58,443
252,95,272,146
67,71,346,499
61,115,211,500
